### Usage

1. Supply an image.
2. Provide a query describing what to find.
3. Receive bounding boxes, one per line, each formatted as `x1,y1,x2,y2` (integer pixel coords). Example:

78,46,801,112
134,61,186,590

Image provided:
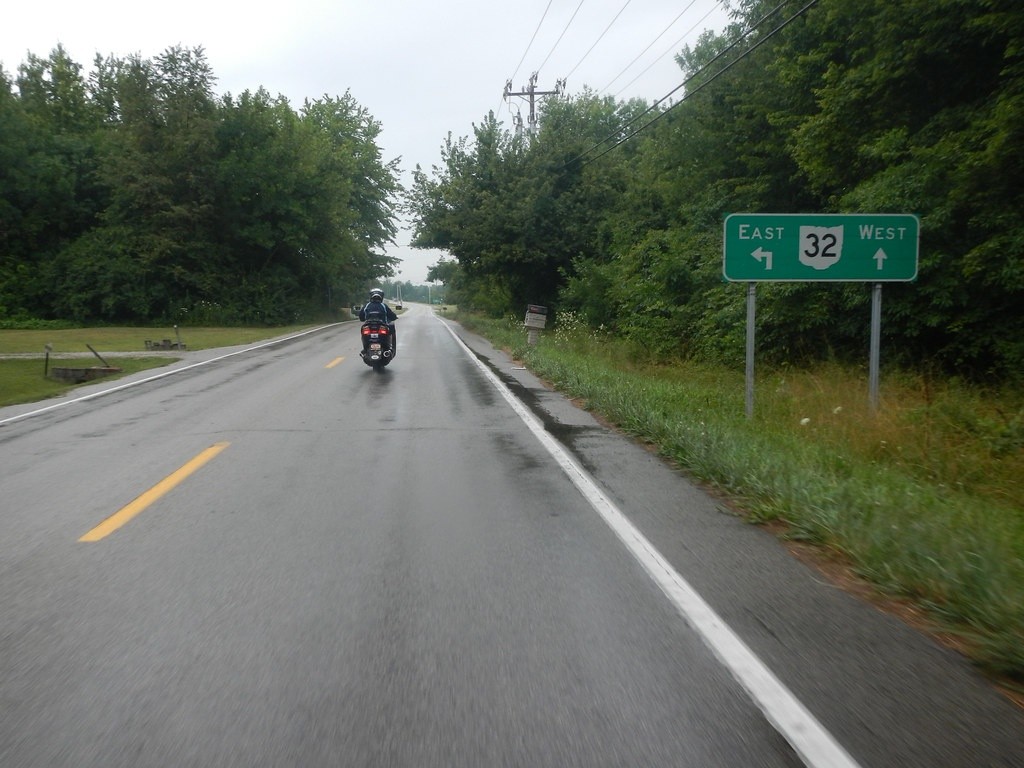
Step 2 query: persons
359,288,397,352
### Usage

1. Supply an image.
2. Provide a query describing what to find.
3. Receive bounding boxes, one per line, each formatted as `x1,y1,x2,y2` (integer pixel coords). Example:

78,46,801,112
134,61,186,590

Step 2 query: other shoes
361,350,366,354
390,348,393,353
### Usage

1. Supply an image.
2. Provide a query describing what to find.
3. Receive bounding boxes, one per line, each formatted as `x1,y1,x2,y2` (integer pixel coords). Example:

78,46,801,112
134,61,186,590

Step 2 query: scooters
352,303,405,371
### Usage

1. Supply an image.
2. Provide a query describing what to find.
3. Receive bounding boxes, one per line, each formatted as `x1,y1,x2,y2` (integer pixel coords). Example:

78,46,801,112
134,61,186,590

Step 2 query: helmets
370,289,384,299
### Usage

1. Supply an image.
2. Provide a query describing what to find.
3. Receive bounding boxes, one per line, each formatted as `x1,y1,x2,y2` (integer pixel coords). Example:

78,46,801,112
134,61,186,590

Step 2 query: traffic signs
722,212,921,284
